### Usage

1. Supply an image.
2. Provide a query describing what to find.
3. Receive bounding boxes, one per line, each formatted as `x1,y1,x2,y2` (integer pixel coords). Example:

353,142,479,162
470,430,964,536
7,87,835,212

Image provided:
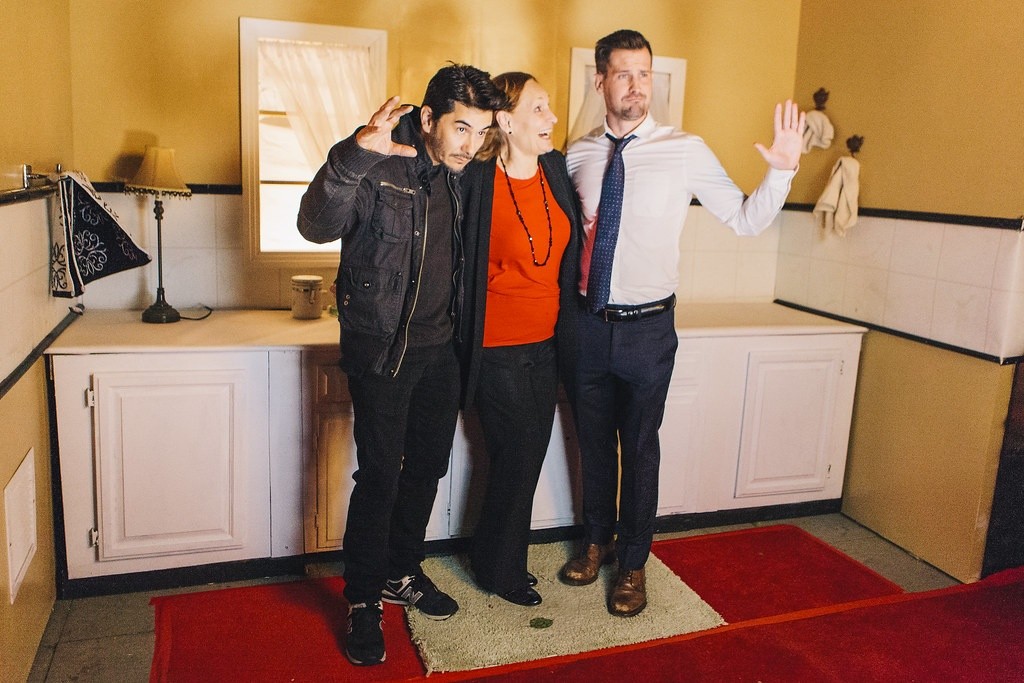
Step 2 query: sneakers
381,566,459,621
609,567,646,616
562,537,616,585
343,594,386,665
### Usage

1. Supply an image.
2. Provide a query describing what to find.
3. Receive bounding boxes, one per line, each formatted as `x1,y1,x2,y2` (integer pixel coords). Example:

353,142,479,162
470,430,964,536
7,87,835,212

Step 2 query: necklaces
498,152,553,267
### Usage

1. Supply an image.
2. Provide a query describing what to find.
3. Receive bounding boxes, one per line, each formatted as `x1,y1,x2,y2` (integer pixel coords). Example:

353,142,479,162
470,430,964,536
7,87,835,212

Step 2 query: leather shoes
497,572,542,605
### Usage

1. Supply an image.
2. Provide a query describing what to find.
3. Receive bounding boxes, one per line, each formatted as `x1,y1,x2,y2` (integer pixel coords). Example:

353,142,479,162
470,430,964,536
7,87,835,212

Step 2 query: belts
579,292,676,321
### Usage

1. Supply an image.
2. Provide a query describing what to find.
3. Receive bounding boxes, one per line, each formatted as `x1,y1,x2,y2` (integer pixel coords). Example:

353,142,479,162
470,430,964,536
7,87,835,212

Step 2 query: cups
290,275,323,320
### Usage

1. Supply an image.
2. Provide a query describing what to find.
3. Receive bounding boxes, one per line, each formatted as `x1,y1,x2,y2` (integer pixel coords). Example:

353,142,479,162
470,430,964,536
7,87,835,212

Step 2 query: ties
586,133,636,314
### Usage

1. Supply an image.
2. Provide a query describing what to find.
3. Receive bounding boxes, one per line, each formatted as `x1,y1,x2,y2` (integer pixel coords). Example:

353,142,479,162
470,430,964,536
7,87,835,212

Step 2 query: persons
463,72,584,606
296,62,513,665
558,29,812,616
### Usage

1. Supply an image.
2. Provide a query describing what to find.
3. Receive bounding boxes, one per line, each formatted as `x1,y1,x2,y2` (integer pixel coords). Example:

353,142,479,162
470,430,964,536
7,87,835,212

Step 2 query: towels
799,109,835,153
51,169,154,298
811,154,861,245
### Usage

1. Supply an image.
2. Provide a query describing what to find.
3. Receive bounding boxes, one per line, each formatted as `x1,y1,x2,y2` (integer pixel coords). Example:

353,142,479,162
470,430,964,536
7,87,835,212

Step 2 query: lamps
124,144,192,323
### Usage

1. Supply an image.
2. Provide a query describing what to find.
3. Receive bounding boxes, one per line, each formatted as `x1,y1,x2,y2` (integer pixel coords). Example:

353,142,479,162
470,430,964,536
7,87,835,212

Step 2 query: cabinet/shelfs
40,300,870,601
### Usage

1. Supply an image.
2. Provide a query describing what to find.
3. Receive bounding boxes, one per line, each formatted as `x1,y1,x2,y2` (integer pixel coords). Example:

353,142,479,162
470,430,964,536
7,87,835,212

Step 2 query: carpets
401,533,729,677
